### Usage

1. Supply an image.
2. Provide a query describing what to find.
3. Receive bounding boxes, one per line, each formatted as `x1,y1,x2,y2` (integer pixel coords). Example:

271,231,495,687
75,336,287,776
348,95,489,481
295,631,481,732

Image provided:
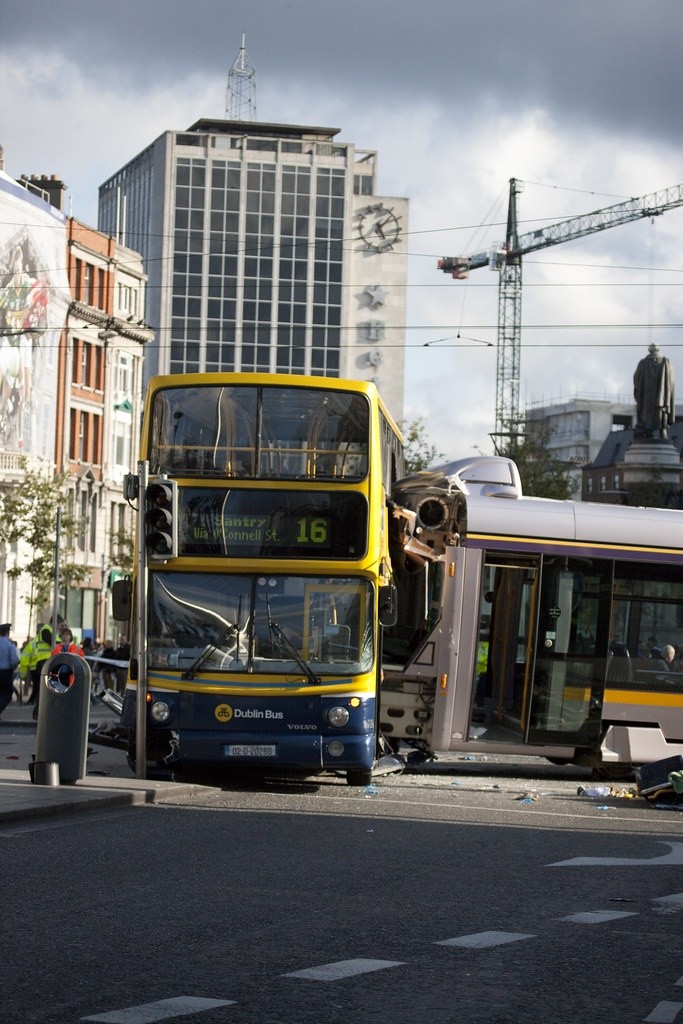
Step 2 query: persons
0,614,132,719
578,636,683,690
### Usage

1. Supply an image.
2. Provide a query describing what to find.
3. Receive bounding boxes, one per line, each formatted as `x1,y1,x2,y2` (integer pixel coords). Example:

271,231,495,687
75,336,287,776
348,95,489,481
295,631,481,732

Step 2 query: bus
112,370,410,792
381,456,683,804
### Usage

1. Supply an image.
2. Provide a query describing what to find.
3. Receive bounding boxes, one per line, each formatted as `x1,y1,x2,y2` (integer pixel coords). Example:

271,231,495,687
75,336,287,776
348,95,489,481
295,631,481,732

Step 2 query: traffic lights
139,477,181,560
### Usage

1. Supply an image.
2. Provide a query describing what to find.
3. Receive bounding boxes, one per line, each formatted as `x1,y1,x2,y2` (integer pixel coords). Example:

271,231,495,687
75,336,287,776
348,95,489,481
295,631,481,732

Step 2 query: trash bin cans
33,652,92,786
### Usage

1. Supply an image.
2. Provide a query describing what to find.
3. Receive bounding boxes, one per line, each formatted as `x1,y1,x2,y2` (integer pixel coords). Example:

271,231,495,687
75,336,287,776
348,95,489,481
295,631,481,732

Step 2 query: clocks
357,202,405,253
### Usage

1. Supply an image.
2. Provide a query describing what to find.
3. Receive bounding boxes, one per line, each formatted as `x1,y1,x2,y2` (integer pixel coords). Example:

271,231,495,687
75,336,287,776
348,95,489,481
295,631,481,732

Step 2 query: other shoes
24,700,34,705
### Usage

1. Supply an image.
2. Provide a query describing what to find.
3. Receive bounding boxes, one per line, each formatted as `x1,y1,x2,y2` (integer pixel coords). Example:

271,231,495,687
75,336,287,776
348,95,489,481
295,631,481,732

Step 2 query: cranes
436,175,682,474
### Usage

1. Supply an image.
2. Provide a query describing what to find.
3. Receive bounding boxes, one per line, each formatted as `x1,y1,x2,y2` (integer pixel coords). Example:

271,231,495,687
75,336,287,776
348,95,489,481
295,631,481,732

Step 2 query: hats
0,624,11,631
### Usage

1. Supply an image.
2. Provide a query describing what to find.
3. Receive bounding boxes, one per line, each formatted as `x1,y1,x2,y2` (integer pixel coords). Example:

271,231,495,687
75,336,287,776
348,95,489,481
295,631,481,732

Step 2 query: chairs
577,635,676,683
